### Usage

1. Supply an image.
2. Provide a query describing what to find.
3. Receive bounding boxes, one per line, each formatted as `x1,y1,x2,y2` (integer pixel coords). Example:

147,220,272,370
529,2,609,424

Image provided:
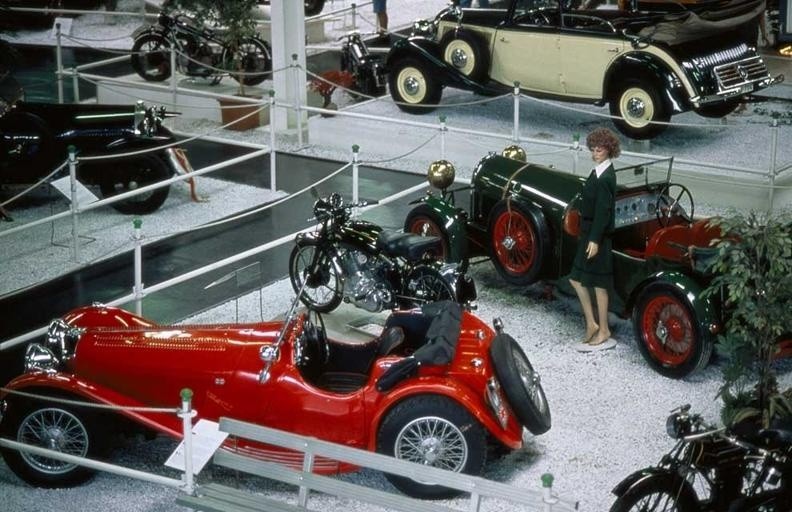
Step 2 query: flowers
311,70,357,108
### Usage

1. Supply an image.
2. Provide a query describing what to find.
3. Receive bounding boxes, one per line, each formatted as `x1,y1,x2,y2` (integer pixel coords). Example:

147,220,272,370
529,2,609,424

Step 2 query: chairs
625,224,688,261
688,215,725,249
322,325,406,394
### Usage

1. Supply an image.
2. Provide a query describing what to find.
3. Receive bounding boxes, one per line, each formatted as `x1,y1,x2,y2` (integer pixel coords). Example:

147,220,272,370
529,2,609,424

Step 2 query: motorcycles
130,1,272,86
289,185,477,313
610,403,792,511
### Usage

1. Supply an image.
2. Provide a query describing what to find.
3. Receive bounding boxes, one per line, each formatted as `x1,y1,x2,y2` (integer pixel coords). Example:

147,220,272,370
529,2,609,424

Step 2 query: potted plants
688,204,792,449
160,0,264,131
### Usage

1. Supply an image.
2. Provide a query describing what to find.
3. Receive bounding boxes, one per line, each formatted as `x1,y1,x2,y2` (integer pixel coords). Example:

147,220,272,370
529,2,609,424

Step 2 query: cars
404,145,792,378
0,73,181,215
386,1,785,139
0,298,552,499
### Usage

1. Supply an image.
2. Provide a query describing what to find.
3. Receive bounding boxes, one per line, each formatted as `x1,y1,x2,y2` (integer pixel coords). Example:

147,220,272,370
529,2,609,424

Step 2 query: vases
322,103,337,118
